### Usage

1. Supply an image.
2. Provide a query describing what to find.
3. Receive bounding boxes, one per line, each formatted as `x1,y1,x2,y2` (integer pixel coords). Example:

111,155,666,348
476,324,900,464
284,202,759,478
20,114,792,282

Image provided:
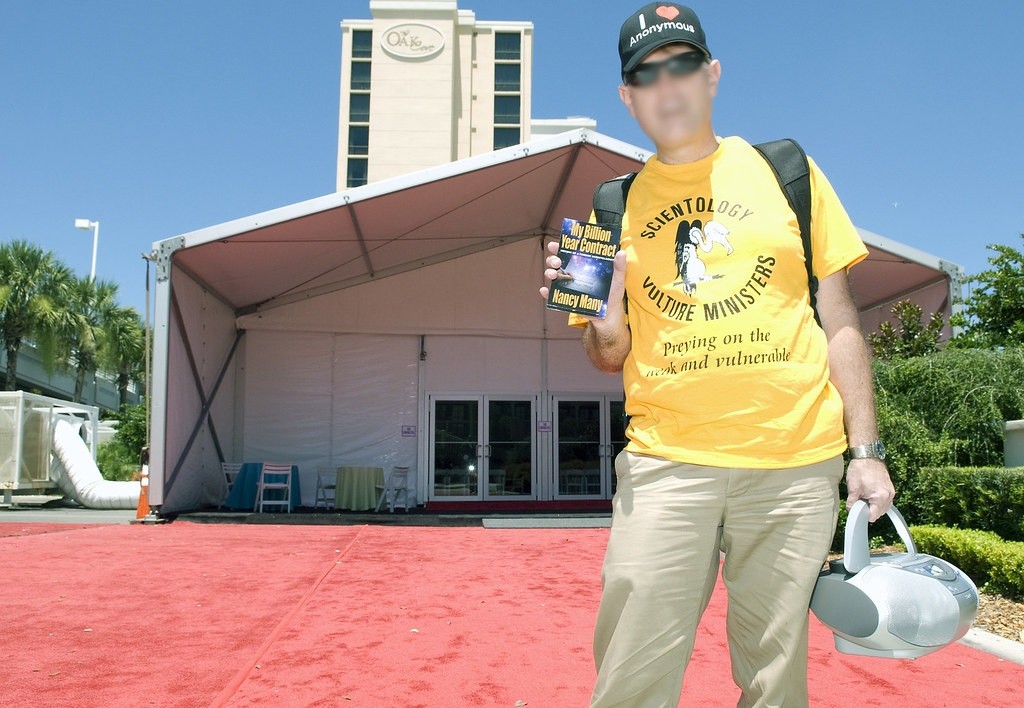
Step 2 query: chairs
316,466,336,510
560,469,600,494
489,469,506,495
374,466,410,512
217,461,244,512
254,461,293,513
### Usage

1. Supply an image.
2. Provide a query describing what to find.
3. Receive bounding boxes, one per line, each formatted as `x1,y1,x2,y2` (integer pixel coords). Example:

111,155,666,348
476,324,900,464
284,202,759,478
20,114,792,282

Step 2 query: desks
224,462,301,513
333,467,387,511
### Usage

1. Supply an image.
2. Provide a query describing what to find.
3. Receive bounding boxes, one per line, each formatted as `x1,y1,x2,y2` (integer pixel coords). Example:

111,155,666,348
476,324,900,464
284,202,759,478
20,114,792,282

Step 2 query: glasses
623,51,709,87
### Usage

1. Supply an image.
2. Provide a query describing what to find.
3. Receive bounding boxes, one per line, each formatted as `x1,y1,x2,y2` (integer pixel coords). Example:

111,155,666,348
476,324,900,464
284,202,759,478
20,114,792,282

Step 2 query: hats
618,1,712,86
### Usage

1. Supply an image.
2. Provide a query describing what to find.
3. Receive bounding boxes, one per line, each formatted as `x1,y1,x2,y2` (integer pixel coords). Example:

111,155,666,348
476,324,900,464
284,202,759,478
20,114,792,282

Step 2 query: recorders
809,499,978,660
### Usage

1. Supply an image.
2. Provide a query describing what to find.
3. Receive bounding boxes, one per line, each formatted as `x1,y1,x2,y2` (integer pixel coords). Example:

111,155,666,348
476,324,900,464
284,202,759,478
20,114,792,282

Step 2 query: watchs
849,440,885,460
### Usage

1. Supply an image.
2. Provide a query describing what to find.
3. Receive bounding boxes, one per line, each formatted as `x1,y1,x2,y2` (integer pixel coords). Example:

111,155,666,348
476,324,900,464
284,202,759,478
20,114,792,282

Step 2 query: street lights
75,218,100,286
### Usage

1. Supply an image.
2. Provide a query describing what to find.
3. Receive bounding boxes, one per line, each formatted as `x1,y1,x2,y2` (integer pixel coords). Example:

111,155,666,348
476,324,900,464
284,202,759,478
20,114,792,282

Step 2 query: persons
540,1,895,708
